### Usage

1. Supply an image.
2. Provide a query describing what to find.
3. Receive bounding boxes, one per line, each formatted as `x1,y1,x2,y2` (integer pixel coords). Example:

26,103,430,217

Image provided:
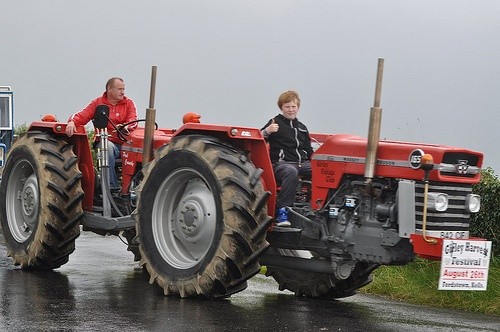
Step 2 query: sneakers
275,209,291,227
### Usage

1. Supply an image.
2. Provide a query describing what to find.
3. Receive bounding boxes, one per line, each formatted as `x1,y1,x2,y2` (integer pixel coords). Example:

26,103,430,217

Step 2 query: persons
259,90,316,227
65,77,139,198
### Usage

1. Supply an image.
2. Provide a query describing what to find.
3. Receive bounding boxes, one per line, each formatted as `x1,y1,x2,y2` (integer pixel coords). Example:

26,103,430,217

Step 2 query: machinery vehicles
0,58,487,301
